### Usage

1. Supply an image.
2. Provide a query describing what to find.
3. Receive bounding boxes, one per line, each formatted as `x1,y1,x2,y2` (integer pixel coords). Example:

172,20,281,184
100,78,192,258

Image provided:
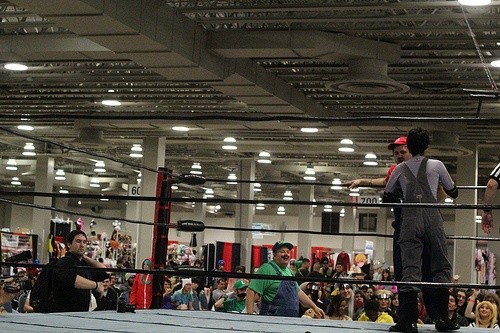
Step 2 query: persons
332,261,428,325
89,219,98,238
74,218,85,231
51,214,73,236
482,163,500,235
189,231,198,248
30,229,105,312
289,256,334,320
343,128,460,333
162,248,215,311
92,254,153,310
211,260,261,313
448,274,500,333
336,248,352,275
111,220,123,243
1,266,41,313
247,240,326,319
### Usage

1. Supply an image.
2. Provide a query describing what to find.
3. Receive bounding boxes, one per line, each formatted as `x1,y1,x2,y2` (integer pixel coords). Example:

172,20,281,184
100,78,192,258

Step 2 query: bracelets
468,299,476,303
94,282,98,290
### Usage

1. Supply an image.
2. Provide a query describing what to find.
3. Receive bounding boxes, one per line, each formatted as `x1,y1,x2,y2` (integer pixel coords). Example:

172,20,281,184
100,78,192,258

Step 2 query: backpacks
30,258,71,313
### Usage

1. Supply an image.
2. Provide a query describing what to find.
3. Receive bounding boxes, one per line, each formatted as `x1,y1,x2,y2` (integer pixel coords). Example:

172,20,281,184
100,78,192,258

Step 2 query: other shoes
435,321,460,331
390,321,418,333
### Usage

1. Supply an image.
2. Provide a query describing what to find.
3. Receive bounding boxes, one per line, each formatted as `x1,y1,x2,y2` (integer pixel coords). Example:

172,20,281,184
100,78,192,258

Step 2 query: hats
180,278,193,294
388,136,407,150
273,240,293,253
233,281,248,290
